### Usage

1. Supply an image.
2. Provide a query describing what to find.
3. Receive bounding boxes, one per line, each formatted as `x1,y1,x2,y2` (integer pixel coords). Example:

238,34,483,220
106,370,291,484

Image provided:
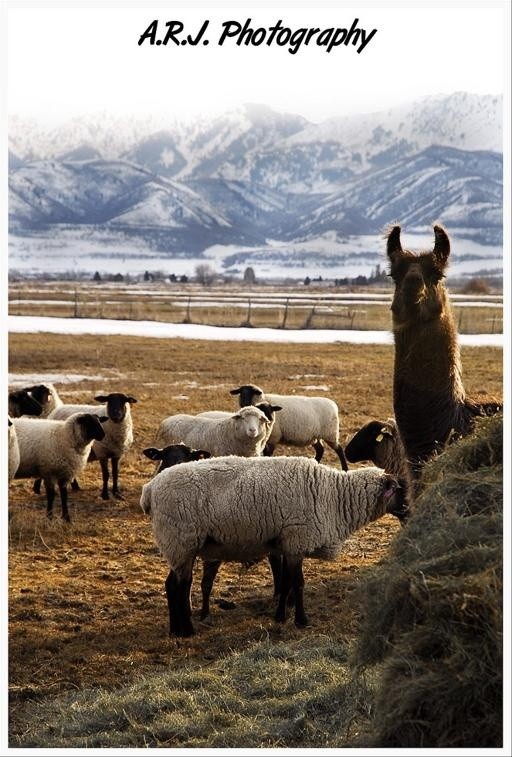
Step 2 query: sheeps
8,381,135,525
138,382,407,640
